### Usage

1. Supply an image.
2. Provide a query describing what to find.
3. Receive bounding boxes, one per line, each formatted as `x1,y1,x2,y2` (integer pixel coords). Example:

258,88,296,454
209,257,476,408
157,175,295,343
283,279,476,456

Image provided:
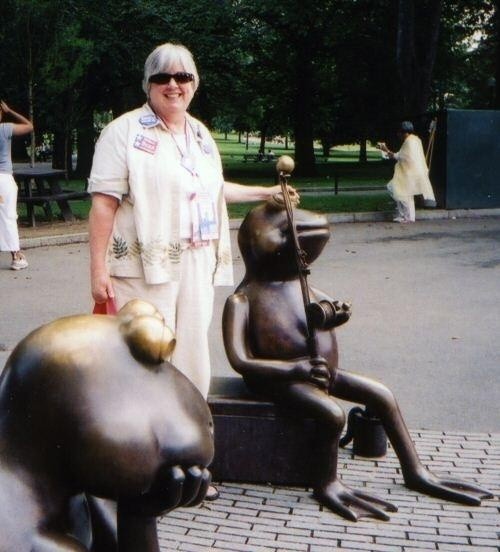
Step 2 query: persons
0,99,35,272
375,120,436,223
255,148,264,161
268,147,276,161
84,42,303,502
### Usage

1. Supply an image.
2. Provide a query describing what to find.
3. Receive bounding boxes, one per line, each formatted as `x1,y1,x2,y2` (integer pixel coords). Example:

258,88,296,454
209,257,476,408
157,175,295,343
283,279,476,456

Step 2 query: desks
13,168,76,227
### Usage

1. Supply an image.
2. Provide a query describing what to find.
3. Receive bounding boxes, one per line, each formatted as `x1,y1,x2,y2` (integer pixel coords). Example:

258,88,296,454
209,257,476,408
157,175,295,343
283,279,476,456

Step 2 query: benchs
28,190,91,221
243,154,281,165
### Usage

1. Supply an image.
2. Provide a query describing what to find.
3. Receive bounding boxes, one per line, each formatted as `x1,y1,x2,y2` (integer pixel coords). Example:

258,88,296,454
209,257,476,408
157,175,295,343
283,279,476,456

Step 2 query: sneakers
12,254,28,270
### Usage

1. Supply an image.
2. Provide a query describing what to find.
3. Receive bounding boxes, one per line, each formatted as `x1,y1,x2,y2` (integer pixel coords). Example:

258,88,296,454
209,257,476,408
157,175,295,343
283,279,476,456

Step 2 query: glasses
148,73,194,84
